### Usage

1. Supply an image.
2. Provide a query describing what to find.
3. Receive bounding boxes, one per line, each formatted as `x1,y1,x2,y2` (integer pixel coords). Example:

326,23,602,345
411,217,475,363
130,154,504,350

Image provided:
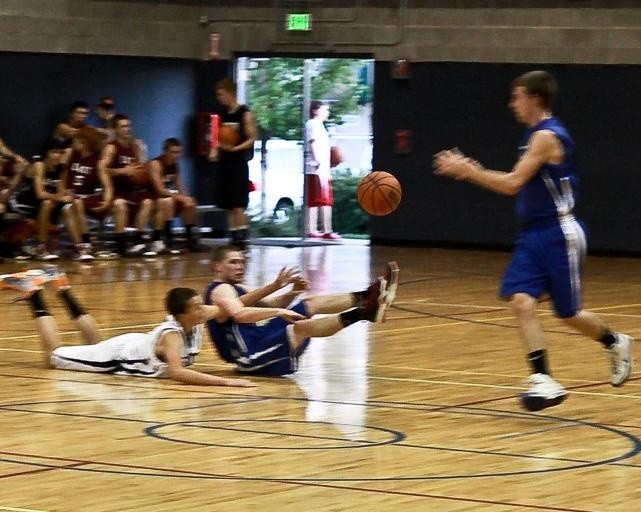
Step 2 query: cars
244,138,303,221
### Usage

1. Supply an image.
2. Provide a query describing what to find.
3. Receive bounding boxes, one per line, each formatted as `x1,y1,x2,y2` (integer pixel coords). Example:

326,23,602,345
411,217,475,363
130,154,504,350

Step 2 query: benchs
1,205,229,256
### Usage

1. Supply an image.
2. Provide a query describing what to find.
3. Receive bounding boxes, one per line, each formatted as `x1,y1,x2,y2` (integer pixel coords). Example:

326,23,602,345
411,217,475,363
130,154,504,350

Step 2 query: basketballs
356,169,402,218
331,146,344,165
218,121,241,144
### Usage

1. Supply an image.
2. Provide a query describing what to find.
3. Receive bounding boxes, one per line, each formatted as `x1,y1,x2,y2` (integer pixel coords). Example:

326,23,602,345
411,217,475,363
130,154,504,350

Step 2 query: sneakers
16,241,210,260
0,264,72,299
606,334,633,386
522,372,569,410
368,261,398,323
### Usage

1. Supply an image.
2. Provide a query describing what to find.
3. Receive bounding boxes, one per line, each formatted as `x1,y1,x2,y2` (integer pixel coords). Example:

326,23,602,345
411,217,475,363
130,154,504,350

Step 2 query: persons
299,100,344,241
1,97,207,257
430,67,636,413
0,264,304,390
204,245,401,376
207,76,256,247
